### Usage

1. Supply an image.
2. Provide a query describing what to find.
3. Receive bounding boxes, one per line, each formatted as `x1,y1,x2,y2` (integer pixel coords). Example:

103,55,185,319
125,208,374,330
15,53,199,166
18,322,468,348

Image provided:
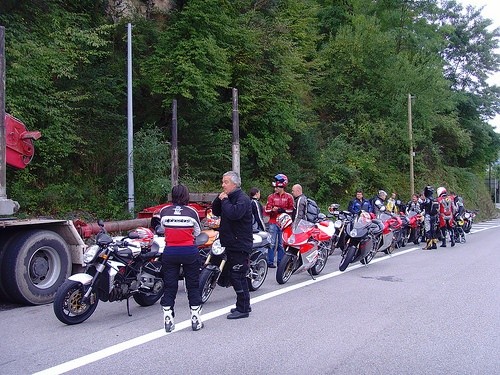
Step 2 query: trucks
0,216,89,308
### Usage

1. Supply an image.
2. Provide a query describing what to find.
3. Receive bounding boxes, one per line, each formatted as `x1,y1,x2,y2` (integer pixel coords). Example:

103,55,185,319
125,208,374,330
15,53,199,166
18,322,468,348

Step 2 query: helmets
424,186,433,196
454,216,464,226
276,213,292,231
437,186,447,197
128,227,154,252
390,216,402,229
312,221,335,241
272,174,288,187
328,204,341,215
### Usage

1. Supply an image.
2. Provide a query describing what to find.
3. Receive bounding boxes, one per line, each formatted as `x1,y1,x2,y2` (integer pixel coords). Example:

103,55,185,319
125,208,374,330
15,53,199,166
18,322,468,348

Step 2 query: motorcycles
176,208,273,305
275,209,336,285
54,219,165,325
328,198,476,272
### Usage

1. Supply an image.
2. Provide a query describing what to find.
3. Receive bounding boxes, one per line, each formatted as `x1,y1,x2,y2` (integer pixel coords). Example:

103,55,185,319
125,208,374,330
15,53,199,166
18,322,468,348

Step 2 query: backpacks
296,195,320,223
369,198,377,206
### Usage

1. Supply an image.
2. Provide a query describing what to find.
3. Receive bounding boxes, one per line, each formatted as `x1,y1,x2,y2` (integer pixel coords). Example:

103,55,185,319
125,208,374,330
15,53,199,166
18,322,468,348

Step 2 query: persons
265,174,295,268
348,190,367,215
160,185,204,331
250,188,265,234
450,191,464,242
386,193,399,215
292,184,306,221
212,171,252,319
436,187,459,247
406,192,425,215
422,186,437,250
372,190,391,216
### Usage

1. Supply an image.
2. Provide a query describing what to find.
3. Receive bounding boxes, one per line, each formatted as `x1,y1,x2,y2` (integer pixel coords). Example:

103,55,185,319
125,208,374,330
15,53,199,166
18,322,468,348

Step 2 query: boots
432,239,437,249
422,239,432,250
190,305,204,331
449,232,457,242
162,306,175,332
440,238,446,247
460,232,466,244
450,235,455,247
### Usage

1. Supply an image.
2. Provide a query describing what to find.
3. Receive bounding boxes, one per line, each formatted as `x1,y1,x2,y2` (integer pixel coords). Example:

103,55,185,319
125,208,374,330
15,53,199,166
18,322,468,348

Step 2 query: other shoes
268,264,276,268
227,307,251,319
